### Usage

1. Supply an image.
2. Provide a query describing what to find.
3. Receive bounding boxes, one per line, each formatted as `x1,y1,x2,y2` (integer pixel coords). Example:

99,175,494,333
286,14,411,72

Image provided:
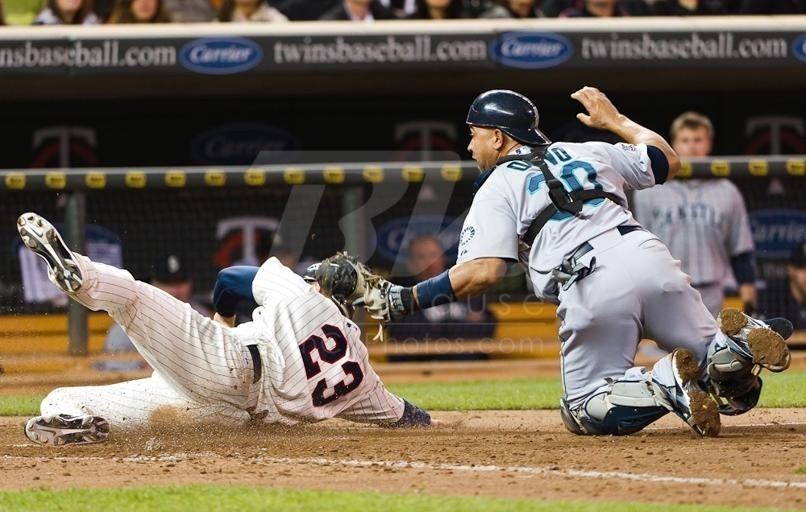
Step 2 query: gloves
353,274,416,326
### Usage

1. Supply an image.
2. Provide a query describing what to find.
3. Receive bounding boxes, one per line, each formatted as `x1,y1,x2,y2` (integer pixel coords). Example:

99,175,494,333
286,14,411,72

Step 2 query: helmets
300,262,321,281
465,89,552,147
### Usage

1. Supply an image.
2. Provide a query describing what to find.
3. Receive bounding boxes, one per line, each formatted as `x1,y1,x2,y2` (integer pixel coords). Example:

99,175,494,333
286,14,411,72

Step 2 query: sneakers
650,348,721,439
715,307,792,373
16,211,84,293
23,413,110,447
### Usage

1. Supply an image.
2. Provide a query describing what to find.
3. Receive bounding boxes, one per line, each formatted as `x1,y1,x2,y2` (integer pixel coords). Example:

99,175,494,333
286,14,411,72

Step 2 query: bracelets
417,270,459,310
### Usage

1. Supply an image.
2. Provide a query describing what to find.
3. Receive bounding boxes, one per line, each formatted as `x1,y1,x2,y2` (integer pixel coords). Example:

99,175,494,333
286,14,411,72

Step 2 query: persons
93,244,212,373
632,111,760,359
557,0,635,18
15,211,443,445
745,241,805,349
12,192,124,310
352,84,795,435
208,228,318,306
383,229,497,362
34,0,172,25
208,0,545,22
